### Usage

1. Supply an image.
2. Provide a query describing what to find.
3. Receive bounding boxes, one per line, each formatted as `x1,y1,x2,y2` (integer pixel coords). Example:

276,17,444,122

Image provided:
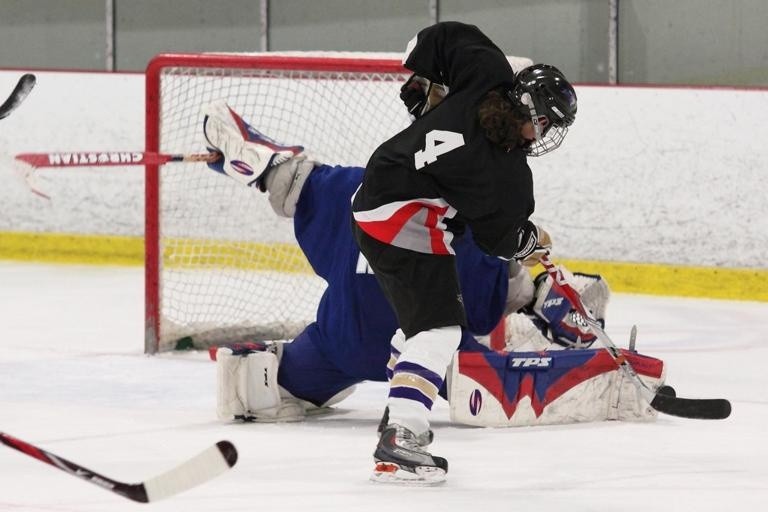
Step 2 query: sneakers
375,408,449,471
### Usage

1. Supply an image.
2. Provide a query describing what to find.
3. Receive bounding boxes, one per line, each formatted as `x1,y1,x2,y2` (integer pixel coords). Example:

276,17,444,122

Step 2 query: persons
350,22,577,486
200,94,667,423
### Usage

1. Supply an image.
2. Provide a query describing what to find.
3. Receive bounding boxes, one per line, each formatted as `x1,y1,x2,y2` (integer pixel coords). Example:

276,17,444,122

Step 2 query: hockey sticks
0,72,36,122
540,257,730,418
0,434,237,504
16,153,222,201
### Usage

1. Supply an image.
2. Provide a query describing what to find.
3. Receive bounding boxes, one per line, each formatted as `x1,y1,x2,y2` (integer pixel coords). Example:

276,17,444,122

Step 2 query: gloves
515,221,549,267
399,73,433,116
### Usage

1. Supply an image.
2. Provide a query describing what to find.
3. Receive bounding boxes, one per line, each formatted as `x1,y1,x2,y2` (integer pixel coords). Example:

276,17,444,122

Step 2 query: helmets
509,62,576,158
